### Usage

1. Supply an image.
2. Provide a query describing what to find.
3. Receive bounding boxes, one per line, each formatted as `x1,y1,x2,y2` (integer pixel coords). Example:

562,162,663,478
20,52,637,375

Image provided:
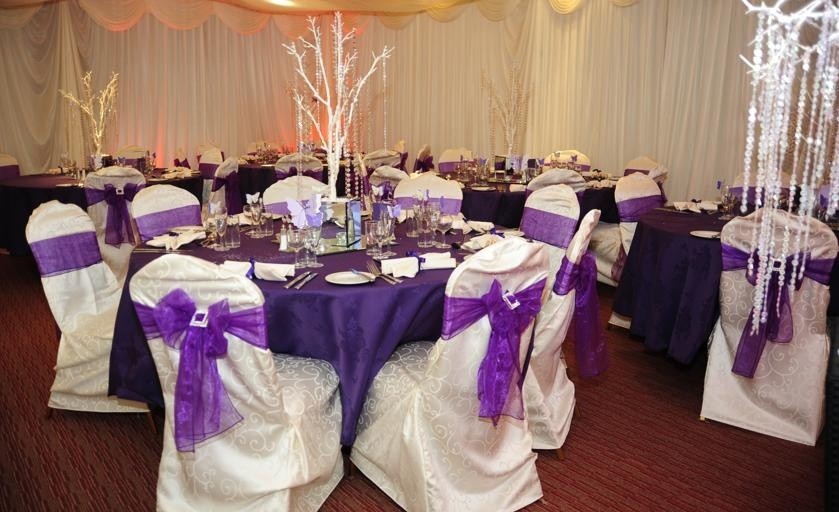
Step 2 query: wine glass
235,140,368,169
57,152,201,183
443,153,603,193
134,195,527,290
717,177,839,231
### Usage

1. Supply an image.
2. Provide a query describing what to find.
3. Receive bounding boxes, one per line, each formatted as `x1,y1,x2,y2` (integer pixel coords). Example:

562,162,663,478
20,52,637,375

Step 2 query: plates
690,230,721,239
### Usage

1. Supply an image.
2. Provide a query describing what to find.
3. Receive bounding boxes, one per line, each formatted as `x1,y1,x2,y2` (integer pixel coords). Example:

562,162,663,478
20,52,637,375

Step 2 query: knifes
654,208,690,215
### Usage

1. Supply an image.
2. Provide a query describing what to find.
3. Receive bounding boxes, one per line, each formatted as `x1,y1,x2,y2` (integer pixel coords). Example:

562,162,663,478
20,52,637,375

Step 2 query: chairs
601,151,839,360
2,141,600,451
129,254,346,511
698,207,838,448
517,209,602,460
349,236,551,511
